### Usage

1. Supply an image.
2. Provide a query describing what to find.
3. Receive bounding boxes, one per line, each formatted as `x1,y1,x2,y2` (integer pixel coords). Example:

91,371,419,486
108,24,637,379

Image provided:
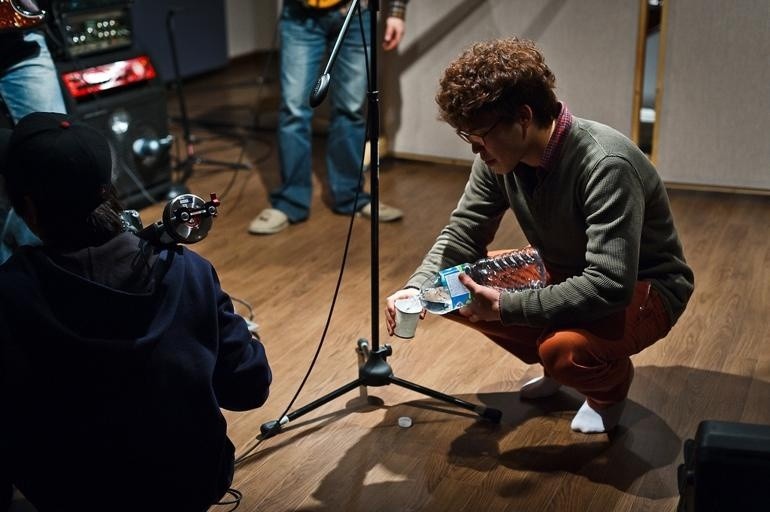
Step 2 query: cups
393,299,423,338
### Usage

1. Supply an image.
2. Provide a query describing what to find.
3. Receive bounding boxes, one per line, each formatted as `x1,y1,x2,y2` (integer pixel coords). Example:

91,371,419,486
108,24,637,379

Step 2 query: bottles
408,247,547,315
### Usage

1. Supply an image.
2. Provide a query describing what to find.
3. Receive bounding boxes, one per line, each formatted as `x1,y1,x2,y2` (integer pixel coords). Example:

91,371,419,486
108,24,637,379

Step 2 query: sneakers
357,202,403,222
249,208,288,235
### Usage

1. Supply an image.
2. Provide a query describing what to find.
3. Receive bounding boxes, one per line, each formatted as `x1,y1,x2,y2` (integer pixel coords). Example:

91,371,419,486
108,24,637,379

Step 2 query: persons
0,1,67,269
0,113,273,510
245,0,408,237
382,34,695,434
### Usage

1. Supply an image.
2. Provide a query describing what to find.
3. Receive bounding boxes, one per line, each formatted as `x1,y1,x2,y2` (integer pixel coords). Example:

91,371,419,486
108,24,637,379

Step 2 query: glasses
456,124,500,147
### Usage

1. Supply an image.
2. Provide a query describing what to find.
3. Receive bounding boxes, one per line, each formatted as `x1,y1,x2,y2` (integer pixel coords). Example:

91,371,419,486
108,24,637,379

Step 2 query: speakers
54,44,173,210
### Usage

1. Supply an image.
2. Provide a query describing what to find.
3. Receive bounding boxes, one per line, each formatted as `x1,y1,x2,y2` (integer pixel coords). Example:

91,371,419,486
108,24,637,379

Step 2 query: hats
9,112,113,216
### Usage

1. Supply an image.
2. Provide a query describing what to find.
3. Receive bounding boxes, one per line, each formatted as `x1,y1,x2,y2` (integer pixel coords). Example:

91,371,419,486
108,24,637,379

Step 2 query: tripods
164,9,255,187
260,0,504,440
